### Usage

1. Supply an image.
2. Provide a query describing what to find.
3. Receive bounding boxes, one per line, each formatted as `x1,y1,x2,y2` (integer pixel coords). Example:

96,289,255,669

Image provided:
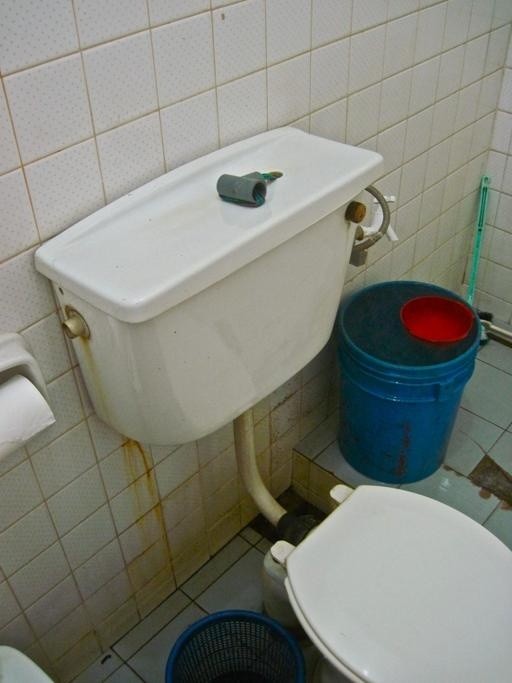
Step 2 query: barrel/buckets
335,278,490,485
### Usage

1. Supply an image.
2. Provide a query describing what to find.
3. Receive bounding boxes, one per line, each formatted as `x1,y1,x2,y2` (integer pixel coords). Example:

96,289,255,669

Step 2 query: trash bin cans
165,609,306,683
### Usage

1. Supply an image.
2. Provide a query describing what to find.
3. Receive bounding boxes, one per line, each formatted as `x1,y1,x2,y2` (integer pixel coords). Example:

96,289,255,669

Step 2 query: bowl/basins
399,294,476,348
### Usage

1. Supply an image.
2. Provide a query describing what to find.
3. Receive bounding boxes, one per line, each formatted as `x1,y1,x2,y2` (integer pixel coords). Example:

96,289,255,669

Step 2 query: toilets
34,126,510,683
